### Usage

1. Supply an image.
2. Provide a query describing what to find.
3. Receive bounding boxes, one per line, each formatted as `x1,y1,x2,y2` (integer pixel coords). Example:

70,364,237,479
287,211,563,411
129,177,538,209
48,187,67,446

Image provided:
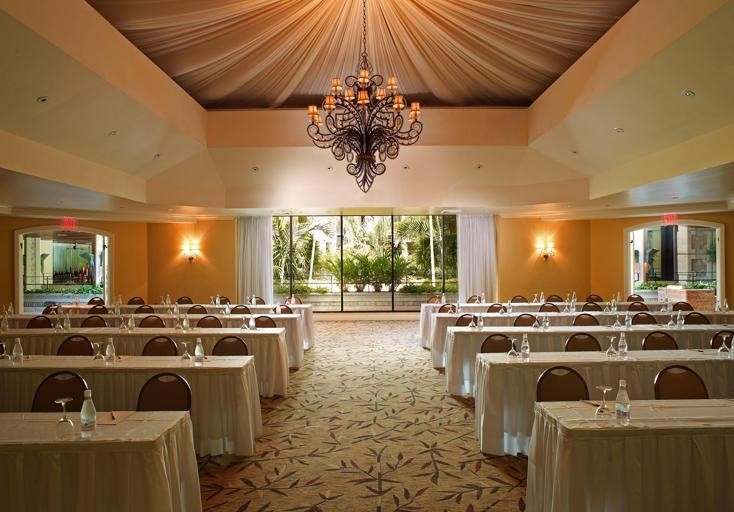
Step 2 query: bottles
249,314,256,330
624,311,632,329
563,299,576,313
128,313,135,332
285,293,296,306
194,337,204,365
1,312,8,331
210,294,220,306
731,337,734,357
566,291,578,302
182,312,189,332
80,389,96,437
498,299,512,315
13,338,23,368
2,302,13,316
269,301,281,316
477,314,483,329
619,332,627,357
106,338,115,362
219,301,230,317
476,293,485,304
448,301,461,314
603,297,617,313
63,313,70,331
435,293,445,304
613,290,620,302
521,333,529,356
543,312,550,327
160,294,171,307
661,298,673,312
108,302,120,316
49,302,63,318
676,310,685,328
167,302,180,316
532,291,544,304
247,294,257,307
613,379,631,425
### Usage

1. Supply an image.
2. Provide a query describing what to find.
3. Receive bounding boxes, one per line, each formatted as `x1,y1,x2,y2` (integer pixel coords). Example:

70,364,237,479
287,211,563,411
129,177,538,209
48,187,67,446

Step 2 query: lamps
305,0,424,194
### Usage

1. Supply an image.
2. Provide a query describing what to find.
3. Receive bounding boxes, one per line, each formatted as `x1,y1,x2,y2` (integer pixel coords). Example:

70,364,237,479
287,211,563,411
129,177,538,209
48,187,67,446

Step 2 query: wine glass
614,313,622,328
241,316,248,332
54,320,63,332
54,397,75,441
119,318,127,330
718,333,730,354
0,342,11,366
180,341,193,362
468,313,475,327
668,313,675,327
532,314,540,327
174,316,182,332
594,385,613,418
605,335,617,356
507,338,518,356
92,342,105,363
